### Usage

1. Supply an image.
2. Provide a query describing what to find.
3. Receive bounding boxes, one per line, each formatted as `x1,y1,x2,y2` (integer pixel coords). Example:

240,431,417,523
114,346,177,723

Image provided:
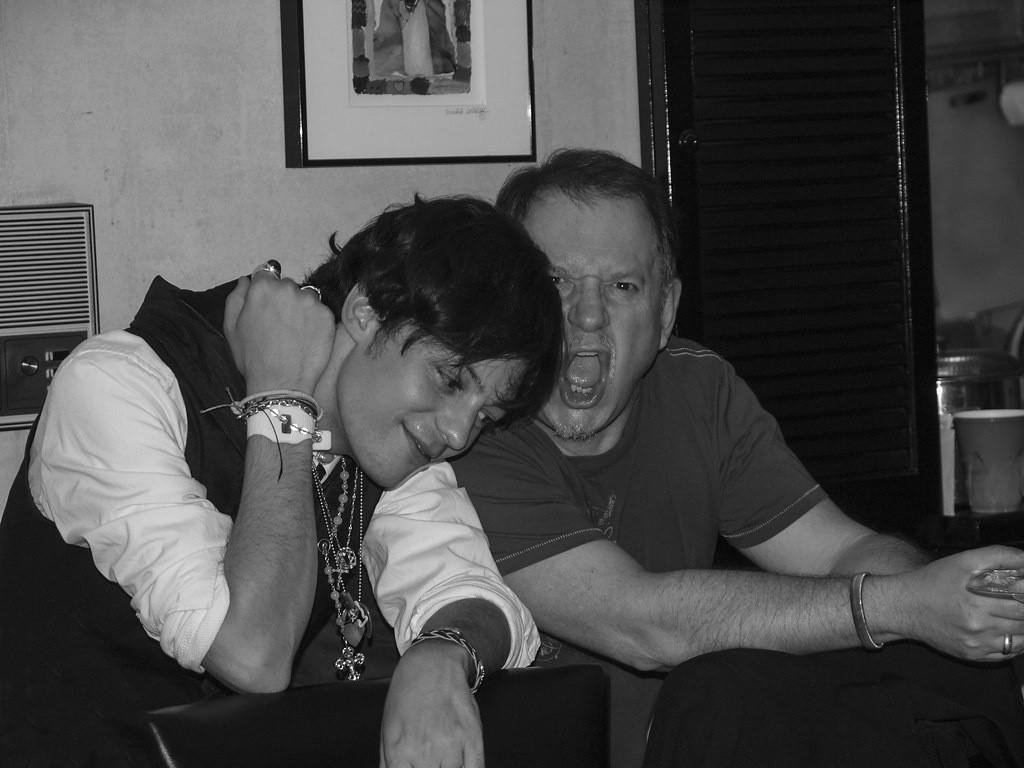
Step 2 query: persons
1,190,540,767
451,144,1023,666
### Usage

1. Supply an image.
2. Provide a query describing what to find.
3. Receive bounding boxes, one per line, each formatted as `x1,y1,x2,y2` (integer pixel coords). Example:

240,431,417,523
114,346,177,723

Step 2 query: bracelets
410,627,486,694
197,385,323,480
850,569,883,653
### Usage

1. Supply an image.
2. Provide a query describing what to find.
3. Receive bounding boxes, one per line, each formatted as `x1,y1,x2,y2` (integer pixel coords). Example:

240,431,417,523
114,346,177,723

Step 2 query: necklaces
308,449,373,682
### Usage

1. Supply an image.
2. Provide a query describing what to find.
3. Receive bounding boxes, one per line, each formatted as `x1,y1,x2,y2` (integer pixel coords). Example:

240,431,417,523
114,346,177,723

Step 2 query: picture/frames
279,0,537,168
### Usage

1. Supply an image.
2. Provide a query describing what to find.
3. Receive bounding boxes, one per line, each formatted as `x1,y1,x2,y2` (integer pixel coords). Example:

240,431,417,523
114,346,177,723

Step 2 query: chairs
128,665,609,768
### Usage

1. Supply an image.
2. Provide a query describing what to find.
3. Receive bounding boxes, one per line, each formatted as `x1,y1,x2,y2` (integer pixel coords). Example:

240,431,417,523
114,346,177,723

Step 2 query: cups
953,408,1024,516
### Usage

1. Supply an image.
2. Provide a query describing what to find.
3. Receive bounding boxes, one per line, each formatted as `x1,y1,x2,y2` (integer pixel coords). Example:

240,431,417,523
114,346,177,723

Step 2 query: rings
1001,634,1011,654
250,259,282,278
301,280,323,301
